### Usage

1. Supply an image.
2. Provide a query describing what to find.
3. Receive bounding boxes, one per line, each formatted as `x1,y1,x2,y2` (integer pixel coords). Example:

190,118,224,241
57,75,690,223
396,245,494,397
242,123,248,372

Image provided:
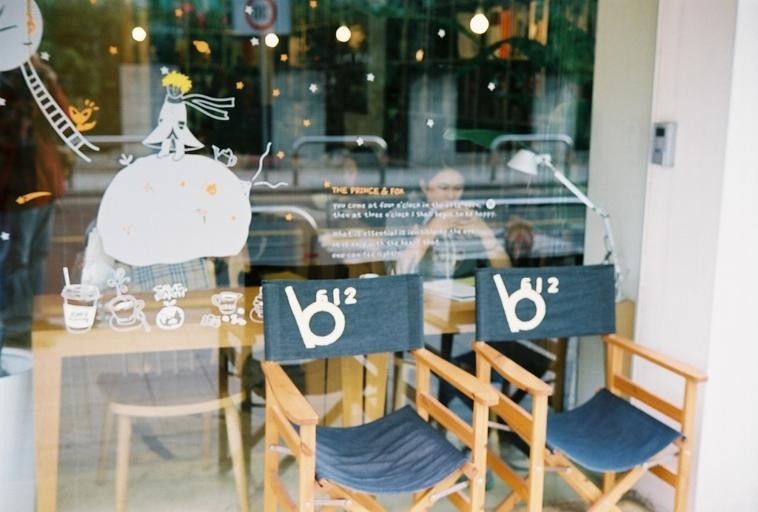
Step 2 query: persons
0,49,73,378
384,156,512,281
327,150,393,225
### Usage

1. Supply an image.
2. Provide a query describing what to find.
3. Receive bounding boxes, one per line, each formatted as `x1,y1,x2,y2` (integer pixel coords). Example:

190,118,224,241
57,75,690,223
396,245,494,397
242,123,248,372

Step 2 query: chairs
479,265,710,509
264,278,495,512
78,210,252,510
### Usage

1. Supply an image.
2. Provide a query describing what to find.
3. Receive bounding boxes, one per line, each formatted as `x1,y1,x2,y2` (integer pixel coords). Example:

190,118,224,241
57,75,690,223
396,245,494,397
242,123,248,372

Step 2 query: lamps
505,148,627,303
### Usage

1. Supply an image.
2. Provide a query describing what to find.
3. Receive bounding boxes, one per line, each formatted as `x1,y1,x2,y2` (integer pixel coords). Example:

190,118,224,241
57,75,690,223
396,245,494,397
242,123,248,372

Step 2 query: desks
27,282,575,510
